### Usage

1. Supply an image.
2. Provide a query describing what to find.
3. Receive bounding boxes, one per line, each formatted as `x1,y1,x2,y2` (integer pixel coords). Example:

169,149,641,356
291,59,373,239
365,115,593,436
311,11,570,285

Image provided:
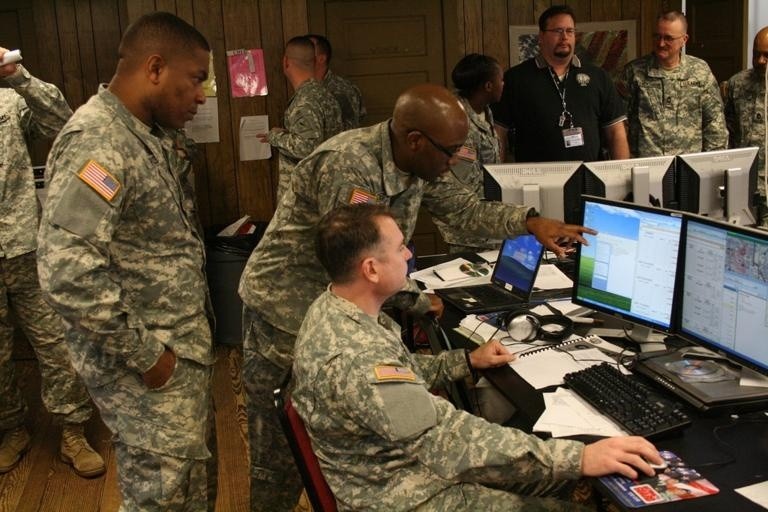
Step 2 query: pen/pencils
433,269,446,284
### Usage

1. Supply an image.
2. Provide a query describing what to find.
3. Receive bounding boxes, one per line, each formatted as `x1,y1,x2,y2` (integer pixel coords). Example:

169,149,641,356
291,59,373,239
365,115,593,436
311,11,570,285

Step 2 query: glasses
411,128,462,157
545,28,575,34
653,33,684,41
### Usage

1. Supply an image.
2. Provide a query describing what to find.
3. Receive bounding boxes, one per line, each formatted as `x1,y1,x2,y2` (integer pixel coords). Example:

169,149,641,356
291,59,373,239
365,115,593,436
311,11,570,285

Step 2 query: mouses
636,456,667,474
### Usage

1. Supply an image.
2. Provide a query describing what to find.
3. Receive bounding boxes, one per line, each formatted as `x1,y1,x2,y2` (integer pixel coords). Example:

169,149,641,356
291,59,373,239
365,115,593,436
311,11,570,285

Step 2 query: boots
56,422,106,478
0,424,33,475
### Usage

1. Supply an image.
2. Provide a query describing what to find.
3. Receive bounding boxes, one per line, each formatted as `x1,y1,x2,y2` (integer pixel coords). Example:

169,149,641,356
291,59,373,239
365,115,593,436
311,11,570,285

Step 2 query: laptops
433,234,546,314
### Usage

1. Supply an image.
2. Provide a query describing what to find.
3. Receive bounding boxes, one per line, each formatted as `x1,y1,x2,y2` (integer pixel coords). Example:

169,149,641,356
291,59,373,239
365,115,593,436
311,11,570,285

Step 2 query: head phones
495,309,574,343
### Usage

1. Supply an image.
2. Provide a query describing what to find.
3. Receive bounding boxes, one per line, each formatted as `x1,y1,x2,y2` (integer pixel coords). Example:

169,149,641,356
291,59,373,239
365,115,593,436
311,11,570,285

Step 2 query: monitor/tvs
582,155,676,209
676,212,768,378
572,194,686,341
483,160,583,224
677,147,759,228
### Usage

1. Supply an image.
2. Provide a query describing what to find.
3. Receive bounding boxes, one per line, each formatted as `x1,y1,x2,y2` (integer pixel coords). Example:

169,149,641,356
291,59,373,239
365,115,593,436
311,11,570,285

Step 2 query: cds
685,367,726,379
459,263,490,278
669,359,717,375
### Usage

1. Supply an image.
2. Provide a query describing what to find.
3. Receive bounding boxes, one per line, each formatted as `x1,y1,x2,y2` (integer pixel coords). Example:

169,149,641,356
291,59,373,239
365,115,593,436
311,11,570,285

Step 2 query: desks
412,249,767,512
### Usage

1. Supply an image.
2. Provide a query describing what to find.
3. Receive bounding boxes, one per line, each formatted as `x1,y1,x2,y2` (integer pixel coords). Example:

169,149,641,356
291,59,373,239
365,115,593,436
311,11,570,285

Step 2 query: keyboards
563,361,692,437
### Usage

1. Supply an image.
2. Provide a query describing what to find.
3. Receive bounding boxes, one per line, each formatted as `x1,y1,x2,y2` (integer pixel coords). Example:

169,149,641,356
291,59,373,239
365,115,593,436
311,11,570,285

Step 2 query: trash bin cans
203,220,269,345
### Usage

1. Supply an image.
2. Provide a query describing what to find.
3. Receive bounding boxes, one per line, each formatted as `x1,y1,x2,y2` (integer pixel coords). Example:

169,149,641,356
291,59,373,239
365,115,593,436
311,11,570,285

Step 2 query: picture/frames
506,16,638,82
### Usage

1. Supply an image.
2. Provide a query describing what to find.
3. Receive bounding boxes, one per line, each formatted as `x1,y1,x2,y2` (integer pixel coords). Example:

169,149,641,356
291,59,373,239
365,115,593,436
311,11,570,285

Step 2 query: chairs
271,364,337,511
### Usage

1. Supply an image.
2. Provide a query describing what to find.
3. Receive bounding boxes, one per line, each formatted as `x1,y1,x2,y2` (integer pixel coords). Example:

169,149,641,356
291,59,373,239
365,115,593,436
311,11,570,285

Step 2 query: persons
34,10,219,512
719,25,768,228
491,5,631,163
286,201,664,512
255,35,345,211
429,53,506,264
615,10,731,159
303,34,367,133
0,46,107,479
236,81,599,511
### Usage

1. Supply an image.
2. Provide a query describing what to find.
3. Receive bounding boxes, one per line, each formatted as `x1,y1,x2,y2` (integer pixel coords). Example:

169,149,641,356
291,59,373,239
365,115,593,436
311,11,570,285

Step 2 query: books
453,302,568,348
498,331,637,390
432,258,489,281
